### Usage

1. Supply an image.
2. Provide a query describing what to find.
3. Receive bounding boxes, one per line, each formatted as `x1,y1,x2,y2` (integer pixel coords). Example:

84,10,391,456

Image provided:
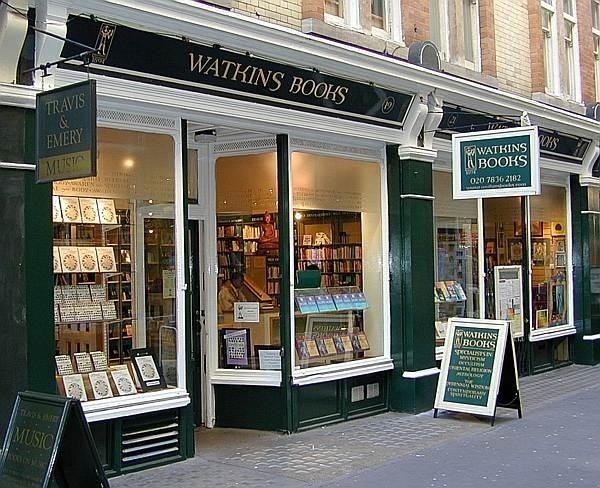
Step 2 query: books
217,224,363,305
56,221,173,358
434,279,467,340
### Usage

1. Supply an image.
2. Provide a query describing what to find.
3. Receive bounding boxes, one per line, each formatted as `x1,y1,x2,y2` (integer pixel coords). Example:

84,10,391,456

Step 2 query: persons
258,210,279,241
217,272,247,324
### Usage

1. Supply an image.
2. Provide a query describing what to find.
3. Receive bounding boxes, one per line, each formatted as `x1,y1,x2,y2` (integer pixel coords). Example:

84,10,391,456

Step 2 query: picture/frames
506,220,568,270
535,308,550,330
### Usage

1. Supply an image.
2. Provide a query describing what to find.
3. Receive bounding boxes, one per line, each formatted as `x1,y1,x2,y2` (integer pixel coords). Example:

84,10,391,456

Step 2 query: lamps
193,128,219,145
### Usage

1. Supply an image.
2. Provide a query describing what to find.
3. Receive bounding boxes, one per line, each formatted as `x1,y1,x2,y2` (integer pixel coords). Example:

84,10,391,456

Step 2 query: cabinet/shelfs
42,215,362,396
